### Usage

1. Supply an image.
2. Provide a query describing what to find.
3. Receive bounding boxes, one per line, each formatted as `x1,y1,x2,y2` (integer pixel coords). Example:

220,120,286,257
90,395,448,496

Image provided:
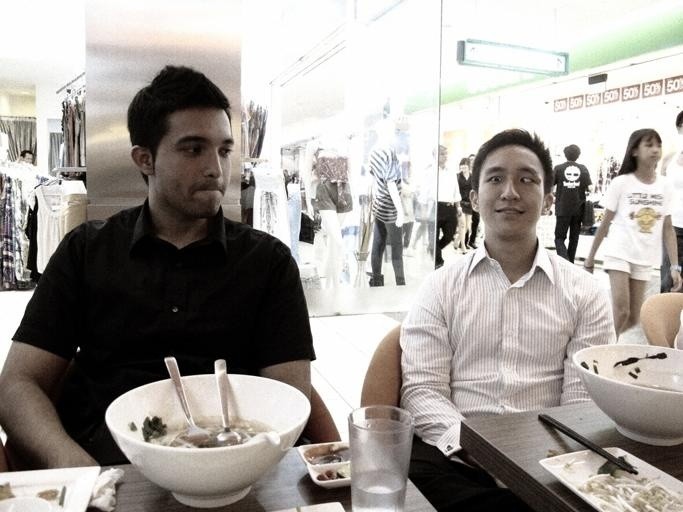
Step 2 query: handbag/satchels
582,200,595,227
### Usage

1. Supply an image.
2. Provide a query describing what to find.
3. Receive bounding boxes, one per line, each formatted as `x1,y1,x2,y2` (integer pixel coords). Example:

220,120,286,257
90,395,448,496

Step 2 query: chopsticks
538,413,638,475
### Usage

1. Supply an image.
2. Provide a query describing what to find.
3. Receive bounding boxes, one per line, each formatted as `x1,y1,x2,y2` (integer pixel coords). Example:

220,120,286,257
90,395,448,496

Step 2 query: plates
299,442,349,489
539,446,682,512
0,464,101,512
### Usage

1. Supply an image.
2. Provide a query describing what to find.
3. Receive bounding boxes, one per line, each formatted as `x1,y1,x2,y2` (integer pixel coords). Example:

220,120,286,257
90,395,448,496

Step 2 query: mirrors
236,0,442,317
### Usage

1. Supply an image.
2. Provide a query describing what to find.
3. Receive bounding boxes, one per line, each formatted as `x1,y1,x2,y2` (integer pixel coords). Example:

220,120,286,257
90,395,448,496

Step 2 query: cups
347,403,414,511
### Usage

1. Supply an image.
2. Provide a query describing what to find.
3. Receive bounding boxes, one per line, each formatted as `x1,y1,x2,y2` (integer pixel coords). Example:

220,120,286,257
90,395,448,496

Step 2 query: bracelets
669,264,683,273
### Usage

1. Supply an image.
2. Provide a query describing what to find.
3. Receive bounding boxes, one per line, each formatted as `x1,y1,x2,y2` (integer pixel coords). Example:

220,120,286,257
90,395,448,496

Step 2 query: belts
438,202,455,208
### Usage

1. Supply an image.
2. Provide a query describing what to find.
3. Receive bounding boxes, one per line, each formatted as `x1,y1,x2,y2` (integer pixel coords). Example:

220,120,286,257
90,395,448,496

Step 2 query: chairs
637,291,682,345
358,324,404,430
299,385,341,446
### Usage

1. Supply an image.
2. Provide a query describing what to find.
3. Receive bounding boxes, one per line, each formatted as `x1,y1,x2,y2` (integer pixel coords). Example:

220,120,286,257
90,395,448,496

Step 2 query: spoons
163,356,209,439
213,358,241,443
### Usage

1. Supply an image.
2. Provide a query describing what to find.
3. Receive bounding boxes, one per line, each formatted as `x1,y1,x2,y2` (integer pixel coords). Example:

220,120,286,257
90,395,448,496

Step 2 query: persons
397,141,478,270
1,63,318,469
584,128,683,338
552,144,594,264
20,149,34,164
366,140,407,286
301,116,360,314
397,128,620,511
660,110,682,296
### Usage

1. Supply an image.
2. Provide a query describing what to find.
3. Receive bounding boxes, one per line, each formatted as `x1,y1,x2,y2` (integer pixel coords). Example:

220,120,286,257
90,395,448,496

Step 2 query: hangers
39,173,83,186
58,76,85,101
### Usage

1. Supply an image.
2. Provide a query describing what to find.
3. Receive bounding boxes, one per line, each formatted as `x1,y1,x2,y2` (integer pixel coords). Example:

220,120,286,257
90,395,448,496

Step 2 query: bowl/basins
104,372,310,509
573,342,682,446
